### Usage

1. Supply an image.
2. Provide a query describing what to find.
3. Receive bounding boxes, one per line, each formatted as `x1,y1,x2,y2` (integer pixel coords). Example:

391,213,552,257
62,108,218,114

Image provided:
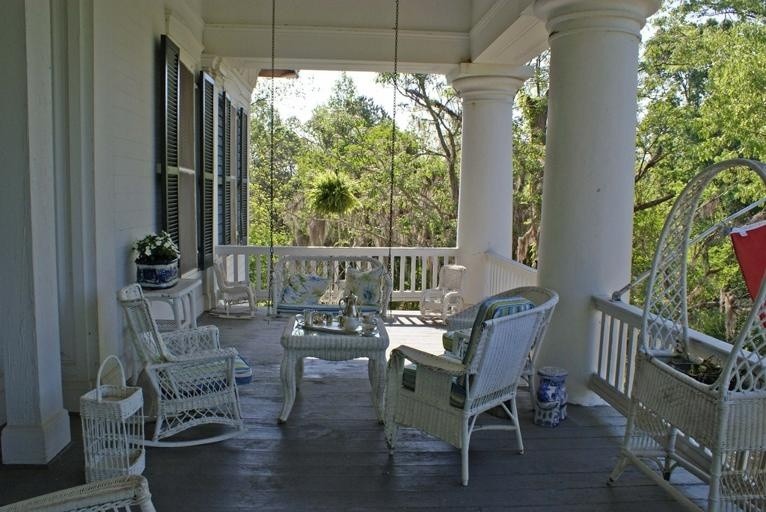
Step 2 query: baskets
79,355,146,484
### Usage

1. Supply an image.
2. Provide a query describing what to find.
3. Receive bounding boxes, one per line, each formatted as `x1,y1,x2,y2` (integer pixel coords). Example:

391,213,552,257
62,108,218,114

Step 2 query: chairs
442,285,560,410
384,307,545,486
118,283,253,448
208,257,256,319
419,264,465,325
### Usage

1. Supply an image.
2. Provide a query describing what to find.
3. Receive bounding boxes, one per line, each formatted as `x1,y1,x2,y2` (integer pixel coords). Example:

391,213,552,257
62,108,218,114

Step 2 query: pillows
456,296,536,389
280,271,331,305
343,263,384,305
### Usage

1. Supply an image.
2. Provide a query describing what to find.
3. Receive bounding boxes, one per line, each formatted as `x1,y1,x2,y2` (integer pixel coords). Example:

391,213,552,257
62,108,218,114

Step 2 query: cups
304,310,313,327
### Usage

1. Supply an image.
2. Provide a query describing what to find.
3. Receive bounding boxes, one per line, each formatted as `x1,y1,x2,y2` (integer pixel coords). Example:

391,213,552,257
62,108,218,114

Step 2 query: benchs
272,255,393,321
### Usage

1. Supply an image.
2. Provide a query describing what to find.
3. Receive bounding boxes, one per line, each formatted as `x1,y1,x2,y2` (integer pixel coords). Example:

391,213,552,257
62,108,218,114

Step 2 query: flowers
132,230,181,264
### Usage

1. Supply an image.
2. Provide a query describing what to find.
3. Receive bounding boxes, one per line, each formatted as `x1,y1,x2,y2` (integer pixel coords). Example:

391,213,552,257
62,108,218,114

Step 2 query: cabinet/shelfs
130,279,202,387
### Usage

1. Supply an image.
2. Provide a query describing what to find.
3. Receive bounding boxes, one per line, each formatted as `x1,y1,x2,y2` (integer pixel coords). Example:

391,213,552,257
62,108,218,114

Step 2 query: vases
136,257,179,290
533,366,568,428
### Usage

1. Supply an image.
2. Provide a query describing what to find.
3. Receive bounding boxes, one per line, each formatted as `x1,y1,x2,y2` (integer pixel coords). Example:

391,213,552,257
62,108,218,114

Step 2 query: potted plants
302,168,363,218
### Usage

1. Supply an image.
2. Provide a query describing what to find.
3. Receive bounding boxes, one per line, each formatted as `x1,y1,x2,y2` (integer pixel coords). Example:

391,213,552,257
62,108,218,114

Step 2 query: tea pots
339,291,361,321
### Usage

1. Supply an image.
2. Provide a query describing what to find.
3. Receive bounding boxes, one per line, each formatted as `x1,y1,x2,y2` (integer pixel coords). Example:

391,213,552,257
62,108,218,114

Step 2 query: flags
729,219,766,327
